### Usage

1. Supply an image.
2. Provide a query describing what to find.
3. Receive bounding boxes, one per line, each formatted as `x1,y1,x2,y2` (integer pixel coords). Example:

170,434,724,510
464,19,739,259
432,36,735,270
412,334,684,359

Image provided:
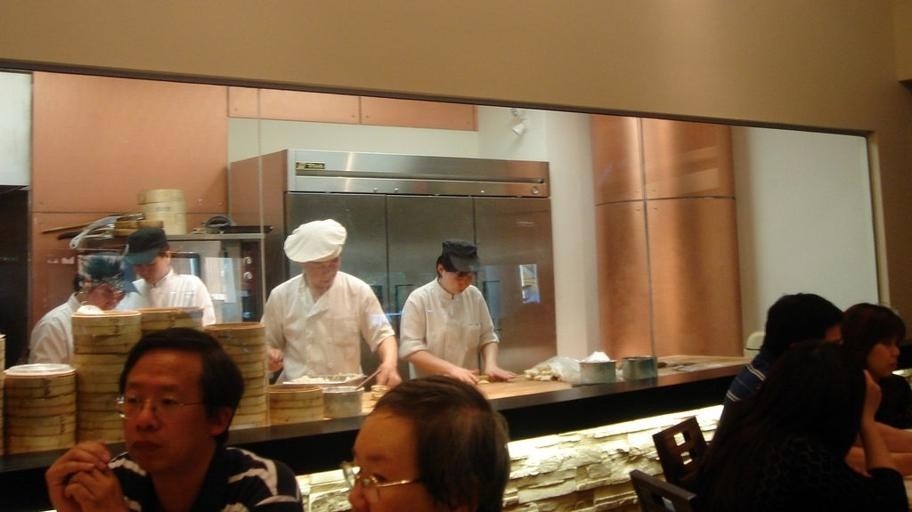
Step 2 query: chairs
628,464,699,512
652,413,712,512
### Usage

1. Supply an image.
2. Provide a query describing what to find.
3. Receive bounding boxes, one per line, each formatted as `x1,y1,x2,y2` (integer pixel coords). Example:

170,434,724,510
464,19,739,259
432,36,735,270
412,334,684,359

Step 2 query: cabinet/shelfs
593,197,745,361
227,82,483,135
587,113,734,205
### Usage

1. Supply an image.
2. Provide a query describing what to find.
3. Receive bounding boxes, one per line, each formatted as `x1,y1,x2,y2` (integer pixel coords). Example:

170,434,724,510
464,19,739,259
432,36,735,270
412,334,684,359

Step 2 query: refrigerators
228,147,558,380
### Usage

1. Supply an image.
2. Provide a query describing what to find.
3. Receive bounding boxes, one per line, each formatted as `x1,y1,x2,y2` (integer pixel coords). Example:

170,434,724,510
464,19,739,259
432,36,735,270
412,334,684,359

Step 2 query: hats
124,226,168,265
442,236,488,274
281,216,349,265
72,250,143,298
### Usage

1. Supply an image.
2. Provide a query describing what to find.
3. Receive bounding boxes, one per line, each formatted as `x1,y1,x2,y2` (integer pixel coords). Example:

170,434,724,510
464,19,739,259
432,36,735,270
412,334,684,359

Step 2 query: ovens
75,223,265,325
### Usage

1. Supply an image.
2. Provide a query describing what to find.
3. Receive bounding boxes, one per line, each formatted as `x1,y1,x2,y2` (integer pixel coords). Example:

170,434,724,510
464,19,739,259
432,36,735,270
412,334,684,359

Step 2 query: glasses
337,462,421,492
111,396,208,420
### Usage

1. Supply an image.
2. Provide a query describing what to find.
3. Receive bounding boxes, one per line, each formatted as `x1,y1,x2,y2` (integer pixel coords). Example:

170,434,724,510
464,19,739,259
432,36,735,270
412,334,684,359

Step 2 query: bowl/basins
578,359,616,387
621,355,657,382
319,385,364,419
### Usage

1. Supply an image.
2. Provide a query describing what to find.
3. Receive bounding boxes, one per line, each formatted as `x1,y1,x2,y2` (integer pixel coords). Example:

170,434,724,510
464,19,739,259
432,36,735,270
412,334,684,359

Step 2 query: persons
399,239,515,386
44,328,303,512
114,227,218,326
26,252,140,363
340,375,511,512
261,219,403,389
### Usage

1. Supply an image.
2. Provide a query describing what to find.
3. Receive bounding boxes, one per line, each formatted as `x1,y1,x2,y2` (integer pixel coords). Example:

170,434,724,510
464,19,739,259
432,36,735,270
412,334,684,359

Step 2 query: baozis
77,304,103,316
